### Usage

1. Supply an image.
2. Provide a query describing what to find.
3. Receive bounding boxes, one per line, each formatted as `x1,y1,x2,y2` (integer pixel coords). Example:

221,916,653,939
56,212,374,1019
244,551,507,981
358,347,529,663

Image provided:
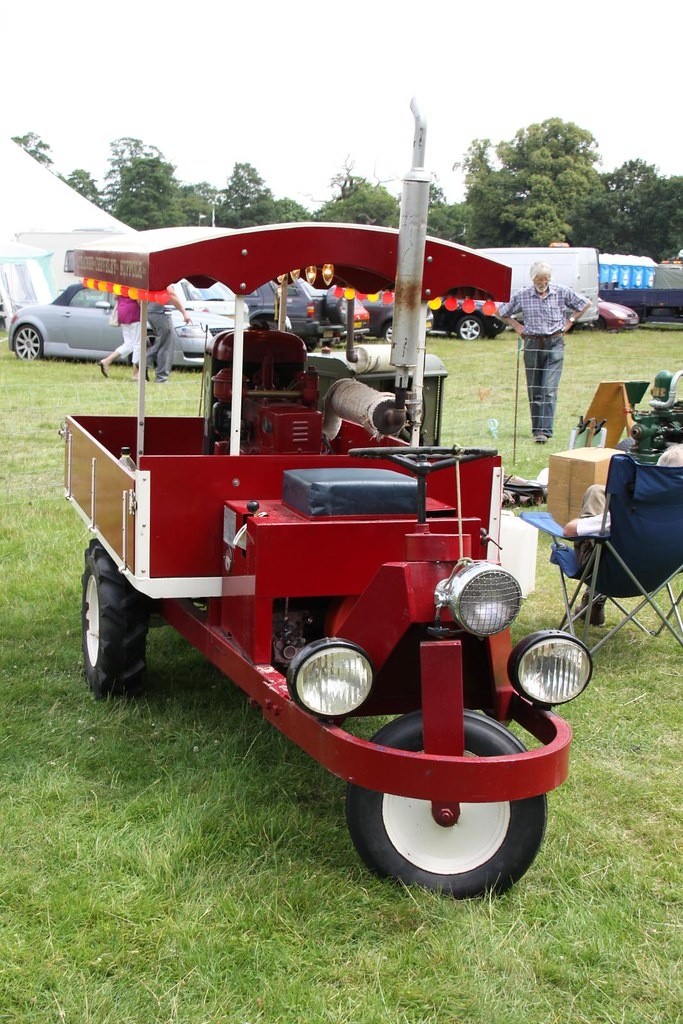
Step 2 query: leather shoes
131,376,138,382
98,359,109,377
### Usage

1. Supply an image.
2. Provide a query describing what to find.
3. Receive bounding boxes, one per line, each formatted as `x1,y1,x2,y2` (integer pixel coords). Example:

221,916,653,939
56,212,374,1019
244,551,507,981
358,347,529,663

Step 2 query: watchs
568,315,576,324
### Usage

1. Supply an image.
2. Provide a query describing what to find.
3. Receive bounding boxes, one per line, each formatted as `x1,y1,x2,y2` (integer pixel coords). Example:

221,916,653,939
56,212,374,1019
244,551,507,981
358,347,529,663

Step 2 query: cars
430,299,506,340
594,297,639,331
341,295,370,336
9,279,234,370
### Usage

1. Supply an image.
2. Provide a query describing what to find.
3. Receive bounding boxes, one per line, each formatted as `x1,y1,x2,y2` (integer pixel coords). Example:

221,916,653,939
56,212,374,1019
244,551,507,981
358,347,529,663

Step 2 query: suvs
363,290,434,343
175,280,250,325
242,277,346,352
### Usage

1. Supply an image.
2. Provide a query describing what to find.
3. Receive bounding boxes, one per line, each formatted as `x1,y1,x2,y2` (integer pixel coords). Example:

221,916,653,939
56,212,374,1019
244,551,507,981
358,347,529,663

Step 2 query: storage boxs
547,447,625,528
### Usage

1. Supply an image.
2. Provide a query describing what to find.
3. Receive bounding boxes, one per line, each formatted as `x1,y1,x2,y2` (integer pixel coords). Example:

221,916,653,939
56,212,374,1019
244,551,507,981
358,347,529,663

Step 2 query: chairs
519,454,683,657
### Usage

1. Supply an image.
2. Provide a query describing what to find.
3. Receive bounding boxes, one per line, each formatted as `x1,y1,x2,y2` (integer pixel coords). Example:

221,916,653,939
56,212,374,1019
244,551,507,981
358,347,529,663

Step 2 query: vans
471,243,599,332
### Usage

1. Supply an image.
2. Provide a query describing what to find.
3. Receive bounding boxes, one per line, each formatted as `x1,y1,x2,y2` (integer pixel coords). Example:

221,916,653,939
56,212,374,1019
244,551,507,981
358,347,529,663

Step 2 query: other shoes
135,360,149,381
536,433,548,443
576,604,602,625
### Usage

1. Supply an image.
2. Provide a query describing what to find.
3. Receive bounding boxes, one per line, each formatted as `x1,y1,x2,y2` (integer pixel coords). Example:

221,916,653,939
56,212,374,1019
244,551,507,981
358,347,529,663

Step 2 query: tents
0,132,137,333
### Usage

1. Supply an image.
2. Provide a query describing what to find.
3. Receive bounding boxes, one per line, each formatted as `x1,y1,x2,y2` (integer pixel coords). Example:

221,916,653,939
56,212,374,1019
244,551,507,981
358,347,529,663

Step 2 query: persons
135,284,193,384
564,444,683,625
495,262,592,442
97,296,148,382
